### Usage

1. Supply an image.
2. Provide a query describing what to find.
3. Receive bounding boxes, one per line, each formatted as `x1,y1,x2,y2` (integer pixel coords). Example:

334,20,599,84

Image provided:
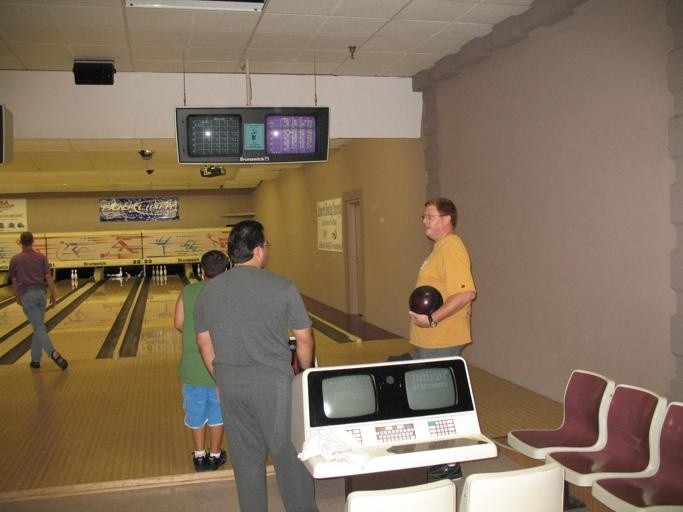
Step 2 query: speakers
73,62,114,85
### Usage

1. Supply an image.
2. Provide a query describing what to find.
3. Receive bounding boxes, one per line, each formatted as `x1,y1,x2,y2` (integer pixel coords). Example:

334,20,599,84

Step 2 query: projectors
200,166,226,178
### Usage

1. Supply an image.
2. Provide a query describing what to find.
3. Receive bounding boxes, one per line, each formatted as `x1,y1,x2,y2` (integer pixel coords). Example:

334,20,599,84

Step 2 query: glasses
263,240,271,248
420,213,445,220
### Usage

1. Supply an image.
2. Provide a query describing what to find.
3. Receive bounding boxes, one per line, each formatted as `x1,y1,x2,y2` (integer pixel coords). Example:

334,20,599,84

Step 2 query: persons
8,231,69,369
407,197,477,483
193,220,318,512
173,249,228,473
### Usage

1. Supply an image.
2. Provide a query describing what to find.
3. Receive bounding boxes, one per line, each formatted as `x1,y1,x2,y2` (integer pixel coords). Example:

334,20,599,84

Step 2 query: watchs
427,314,437,328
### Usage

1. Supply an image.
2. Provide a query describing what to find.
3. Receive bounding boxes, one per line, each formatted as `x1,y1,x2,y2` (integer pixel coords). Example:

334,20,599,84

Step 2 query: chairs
506,368,615,459
591,400,683,511
344,478,457,512
543,383,667,488
457,462,565,512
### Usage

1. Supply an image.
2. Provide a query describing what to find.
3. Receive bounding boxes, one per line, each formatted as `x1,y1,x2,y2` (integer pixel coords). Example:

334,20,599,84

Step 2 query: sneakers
209,450,227,470
30,361,40,368
50,350,68,369
191,451,209,472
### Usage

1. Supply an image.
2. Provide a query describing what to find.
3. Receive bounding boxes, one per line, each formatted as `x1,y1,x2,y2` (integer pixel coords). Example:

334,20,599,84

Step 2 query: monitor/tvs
264,113,319,155
321,373,379,419
404,366,459,411
186,113,243,158
